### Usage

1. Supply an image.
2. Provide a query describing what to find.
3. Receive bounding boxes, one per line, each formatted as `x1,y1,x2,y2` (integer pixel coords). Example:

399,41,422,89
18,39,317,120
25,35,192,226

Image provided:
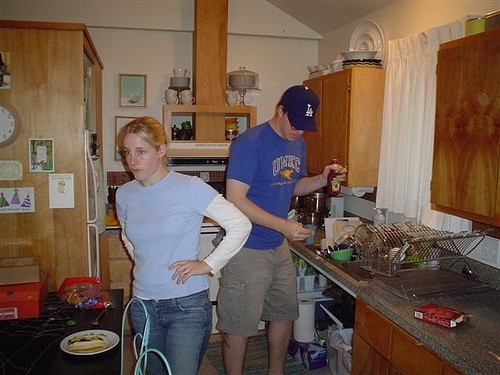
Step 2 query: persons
216,85,347,375
114,116,253,375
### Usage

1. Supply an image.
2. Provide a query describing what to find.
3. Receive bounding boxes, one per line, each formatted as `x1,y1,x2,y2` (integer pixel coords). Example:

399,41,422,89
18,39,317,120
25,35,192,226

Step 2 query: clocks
0,102,22,148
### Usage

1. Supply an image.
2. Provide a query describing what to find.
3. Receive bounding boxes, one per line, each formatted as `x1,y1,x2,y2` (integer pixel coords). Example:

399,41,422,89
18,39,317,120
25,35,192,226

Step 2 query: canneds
388,248,400,276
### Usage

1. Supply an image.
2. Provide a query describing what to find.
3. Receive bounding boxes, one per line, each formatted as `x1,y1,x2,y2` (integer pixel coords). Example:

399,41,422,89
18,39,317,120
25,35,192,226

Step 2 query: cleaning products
372,207,387,226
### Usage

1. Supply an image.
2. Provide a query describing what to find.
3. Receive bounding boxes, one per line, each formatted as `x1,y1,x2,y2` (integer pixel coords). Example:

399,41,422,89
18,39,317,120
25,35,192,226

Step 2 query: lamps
227,66,262,106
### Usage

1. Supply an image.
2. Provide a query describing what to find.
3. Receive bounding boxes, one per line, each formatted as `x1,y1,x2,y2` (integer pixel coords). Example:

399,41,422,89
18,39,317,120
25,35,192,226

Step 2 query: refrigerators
84,129,101,280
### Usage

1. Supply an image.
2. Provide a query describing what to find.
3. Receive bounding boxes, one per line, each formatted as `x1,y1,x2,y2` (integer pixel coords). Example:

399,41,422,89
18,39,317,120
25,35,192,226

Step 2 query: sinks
340,256,418,282
307,243,382,264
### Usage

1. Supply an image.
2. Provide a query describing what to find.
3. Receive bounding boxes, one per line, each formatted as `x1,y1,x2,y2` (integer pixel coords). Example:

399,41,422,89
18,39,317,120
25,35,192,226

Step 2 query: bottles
172,124,180,140
326,159,341,196
373,208,386,226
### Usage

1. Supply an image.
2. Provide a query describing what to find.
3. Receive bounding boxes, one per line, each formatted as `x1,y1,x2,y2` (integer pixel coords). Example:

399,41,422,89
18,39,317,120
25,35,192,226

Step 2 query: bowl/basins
307,60,341,73
330,248,353,261
225,117,239,141
340,50,378,59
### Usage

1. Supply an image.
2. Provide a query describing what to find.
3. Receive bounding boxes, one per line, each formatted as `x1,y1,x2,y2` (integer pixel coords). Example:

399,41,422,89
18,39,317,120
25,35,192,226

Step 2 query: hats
283,85,319,132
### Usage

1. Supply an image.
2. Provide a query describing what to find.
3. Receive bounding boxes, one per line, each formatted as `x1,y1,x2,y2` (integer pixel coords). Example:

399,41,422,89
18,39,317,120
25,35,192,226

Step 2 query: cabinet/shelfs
0,19,105,292
302,67,385,187
431,28,500,226
352,286,500,375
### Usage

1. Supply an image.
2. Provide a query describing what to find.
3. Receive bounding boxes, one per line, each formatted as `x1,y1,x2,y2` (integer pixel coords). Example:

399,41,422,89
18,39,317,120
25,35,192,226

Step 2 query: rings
184,269,187,275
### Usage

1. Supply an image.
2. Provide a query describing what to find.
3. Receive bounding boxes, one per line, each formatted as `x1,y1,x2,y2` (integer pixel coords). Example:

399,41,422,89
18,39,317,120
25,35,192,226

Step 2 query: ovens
199,226,265,334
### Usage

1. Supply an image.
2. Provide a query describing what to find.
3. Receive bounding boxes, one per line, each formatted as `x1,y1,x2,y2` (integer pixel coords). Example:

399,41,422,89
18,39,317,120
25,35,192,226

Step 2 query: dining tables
0,288,124,375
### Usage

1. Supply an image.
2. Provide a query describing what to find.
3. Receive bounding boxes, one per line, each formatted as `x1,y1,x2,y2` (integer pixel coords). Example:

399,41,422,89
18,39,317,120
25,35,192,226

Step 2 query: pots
291,191,326,228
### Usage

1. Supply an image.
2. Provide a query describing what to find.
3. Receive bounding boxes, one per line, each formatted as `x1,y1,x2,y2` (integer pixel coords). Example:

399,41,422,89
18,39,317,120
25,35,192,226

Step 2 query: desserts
67,333,105,352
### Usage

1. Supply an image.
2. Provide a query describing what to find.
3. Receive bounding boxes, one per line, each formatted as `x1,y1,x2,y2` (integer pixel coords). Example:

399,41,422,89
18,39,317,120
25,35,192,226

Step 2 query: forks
90,310,105,326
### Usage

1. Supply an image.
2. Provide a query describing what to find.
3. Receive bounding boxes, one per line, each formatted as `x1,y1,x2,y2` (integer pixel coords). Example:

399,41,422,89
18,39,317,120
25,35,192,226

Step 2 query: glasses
283,111,297,130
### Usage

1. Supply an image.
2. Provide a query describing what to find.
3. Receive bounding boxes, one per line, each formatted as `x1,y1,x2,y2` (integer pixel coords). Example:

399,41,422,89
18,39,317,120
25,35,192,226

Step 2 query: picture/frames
114,116,139,161
29,138,54,173
0,187,36,214
119,73,146,107
48,173,75,208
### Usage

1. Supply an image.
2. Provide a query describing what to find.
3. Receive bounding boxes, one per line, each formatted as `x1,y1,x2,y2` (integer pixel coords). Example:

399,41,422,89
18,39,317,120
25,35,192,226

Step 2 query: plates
341,59,383,69
60,329,119,355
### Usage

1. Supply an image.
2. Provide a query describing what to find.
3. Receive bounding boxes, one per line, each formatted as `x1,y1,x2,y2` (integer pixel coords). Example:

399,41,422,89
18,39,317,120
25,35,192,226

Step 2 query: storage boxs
0,256,50,320
414,303,469,328
287,338,328,371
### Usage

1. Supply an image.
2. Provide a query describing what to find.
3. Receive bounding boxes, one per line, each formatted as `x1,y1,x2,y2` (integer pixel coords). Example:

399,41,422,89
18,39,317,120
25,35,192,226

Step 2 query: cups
173,68,191,77
226,91,240,105
304,224,317,245
164,89,177,104
179,90,193,104
